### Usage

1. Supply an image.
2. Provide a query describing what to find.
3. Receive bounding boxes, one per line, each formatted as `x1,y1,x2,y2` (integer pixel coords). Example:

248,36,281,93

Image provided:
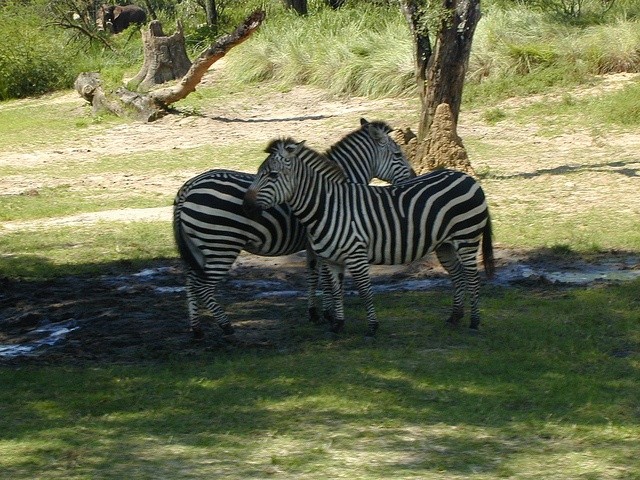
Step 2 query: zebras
173,116,418,343
242,136,496,346
